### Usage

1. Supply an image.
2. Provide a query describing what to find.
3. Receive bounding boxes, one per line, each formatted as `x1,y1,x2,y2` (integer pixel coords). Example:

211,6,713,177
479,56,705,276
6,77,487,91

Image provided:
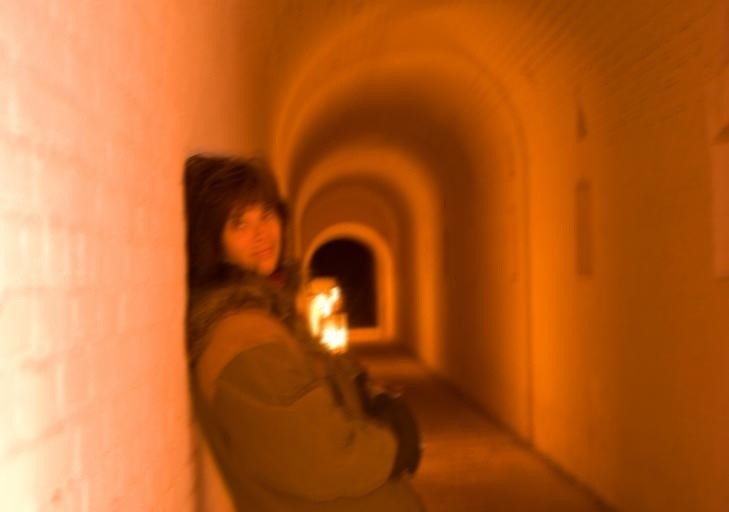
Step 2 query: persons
184,156,425,512
